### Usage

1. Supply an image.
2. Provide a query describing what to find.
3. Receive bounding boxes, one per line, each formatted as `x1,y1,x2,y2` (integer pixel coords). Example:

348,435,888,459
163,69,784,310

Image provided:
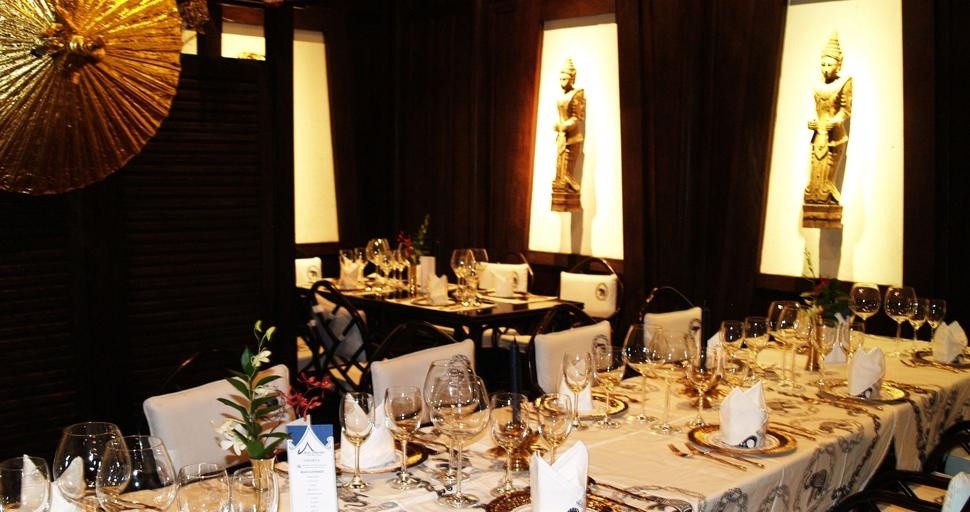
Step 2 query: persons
803,32,852,205
551,59,586,192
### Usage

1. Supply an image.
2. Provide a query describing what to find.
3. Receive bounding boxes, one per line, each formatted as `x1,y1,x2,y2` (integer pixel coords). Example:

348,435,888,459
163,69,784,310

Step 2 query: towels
19,454,87,512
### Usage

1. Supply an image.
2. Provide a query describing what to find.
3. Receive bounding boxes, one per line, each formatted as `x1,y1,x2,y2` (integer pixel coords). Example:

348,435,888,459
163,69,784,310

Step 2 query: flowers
395,213,431,265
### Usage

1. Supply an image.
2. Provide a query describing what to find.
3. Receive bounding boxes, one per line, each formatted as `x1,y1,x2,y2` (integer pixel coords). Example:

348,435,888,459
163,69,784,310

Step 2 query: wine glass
339,238,490,303
0,281,951,512
